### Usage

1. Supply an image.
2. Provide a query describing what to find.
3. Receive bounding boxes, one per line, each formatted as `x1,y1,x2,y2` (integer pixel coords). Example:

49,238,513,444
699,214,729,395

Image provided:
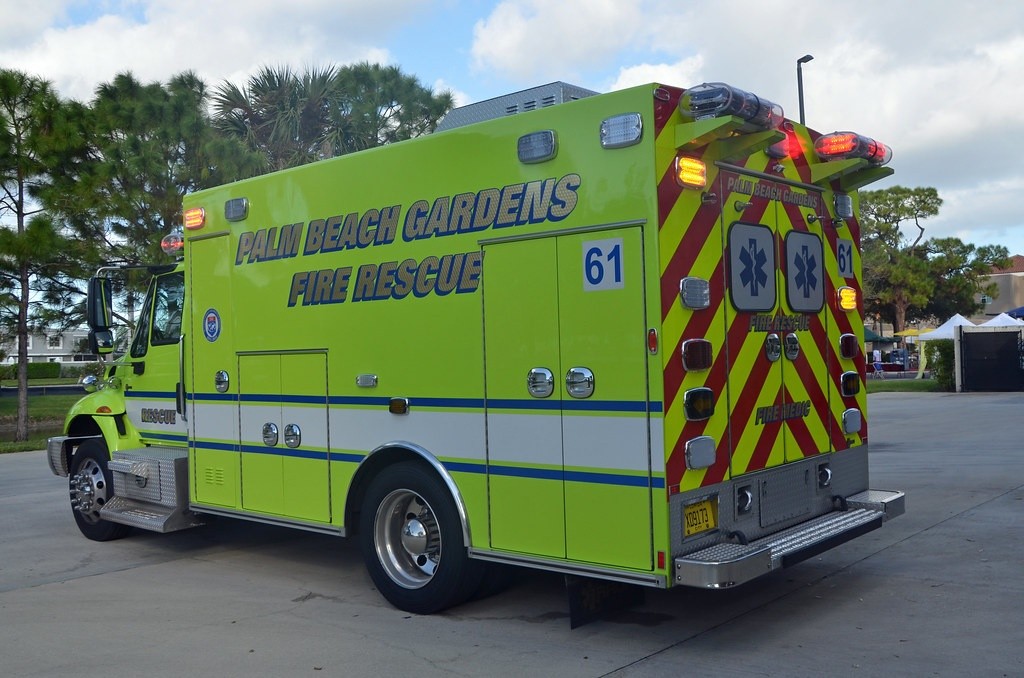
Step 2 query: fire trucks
46,80,907,615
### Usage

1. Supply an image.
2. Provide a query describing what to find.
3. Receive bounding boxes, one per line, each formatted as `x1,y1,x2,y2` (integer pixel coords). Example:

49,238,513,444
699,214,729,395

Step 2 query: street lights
796,54,815,123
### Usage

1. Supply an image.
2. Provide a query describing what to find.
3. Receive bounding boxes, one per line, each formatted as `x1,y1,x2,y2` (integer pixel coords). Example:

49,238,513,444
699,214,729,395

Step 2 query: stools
897,373,905,378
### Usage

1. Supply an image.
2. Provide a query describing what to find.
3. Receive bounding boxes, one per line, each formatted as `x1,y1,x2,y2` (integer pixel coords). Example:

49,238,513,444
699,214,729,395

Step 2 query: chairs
872,362,886,380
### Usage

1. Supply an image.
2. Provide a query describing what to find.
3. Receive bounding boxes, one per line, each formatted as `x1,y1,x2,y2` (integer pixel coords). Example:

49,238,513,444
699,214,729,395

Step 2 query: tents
863,306,1024,380
